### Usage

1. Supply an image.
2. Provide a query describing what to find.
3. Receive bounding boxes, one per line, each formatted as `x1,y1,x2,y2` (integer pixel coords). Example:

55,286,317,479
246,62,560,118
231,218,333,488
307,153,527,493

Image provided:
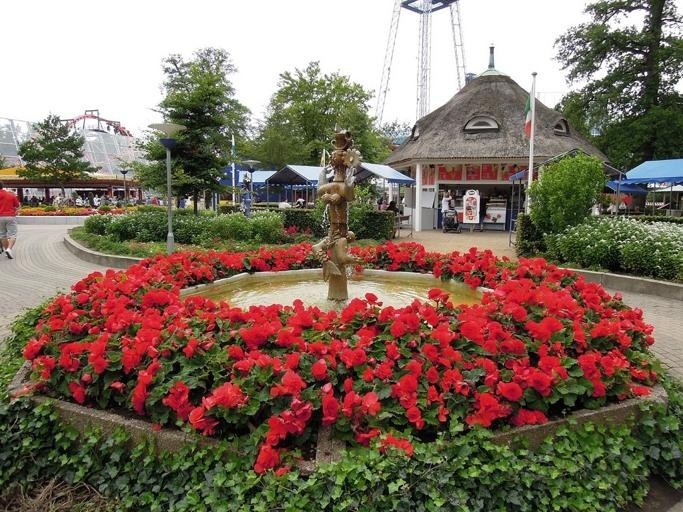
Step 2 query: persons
296,195,305,208
441,192,452,226
0,188,21,261
386,200,403,229
591,201,627,216
22,191,186,209
278,197,299,209
379,200,387,210
377,197,383,210
0,180,3,254
448,190,457,224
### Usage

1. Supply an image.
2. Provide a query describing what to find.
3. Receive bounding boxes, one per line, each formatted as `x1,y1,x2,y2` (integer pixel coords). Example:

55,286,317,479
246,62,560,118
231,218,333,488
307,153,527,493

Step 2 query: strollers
442,210,462,233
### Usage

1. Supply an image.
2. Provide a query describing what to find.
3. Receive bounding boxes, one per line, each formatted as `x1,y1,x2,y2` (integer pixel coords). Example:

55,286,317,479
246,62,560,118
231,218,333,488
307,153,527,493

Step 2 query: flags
523,84,533,139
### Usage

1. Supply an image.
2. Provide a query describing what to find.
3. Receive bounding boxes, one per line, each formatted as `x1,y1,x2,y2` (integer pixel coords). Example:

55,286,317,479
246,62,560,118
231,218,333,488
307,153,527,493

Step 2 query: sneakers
2,248,14,259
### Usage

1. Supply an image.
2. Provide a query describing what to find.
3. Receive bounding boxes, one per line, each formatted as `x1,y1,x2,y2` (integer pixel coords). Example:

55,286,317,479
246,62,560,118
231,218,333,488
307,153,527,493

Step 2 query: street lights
148,122,186,254
120,170,129,209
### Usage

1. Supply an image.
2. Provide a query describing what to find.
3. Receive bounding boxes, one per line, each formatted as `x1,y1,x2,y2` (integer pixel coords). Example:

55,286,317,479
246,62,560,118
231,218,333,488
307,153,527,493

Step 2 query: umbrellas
653,184,683,203
